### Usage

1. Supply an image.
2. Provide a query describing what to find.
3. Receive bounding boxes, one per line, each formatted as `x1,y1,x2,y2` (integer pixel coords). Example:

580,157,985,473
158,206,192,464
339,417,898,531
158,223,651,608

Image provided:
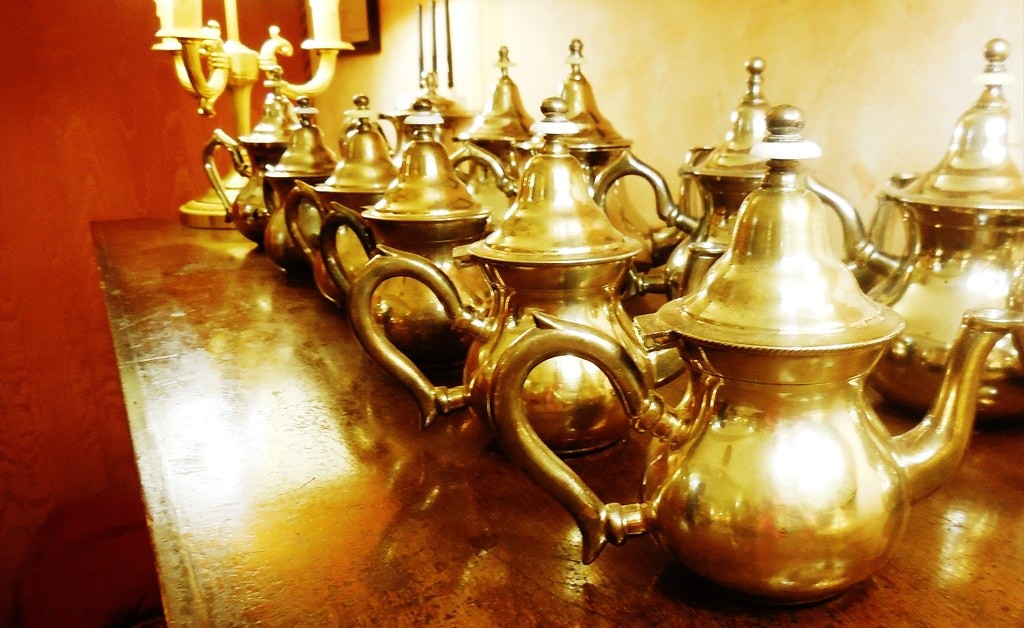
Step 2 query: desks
88,218,1024,628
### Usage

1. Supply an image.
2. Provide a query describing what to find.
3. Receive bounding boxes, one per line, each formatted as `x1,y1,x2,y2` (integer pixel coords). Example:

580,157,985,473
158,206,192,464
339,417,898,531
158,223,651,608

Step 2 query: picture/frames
338,0,380,56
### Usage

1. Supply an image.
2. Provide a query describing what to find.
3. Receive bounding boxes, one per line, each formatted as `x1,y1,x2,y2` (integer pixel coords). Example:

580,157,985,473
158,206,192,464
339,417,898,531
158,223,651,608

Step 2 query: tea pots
201,38,1024,616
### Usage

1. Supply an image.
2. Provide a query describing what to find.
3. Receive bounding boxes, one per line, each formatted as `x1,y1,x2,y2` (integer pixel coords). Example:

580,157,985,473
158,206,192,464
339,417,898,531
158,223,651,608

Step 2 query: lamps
147,0,357,229
